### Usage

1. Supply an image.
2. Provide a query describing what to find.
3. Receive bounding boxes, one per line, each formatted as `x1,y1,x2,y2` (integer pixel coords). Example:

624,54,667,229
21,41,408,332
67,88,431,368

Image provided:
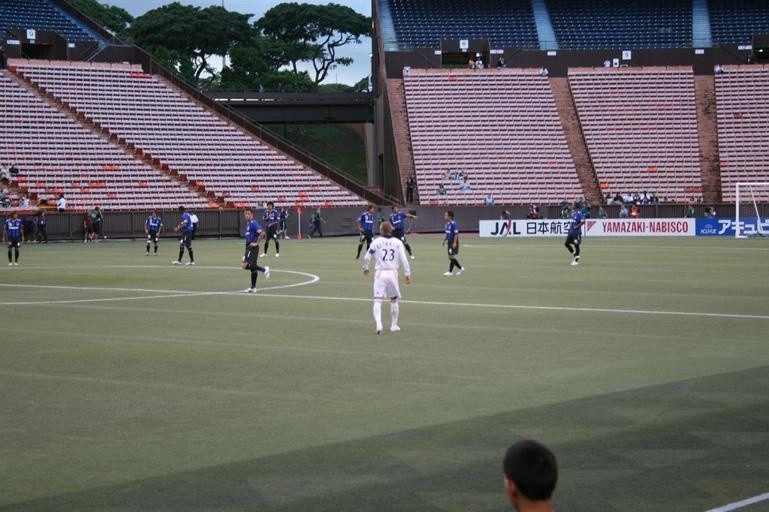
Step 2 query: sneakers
390,327,401,332
260,254,267,257
571,253,579,265
376,327,382,334
276,254,279,257
245,288,256,293
172,261,181,265
264,266,269,279
456,268,464,275
443,272,451,276
185,262,194,265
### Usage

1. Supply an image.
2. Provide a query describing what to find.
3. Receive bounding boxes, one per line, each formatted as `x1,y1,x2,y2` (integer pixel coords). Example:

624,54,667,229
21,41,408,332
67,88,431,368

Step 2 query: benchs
1,0,368,209
388,0,768,205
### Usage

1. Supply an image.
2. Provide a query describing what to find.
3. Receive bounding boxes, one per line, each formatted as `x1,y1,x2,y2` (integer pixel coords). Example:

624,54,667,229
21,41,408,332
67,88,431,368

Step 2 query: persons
439,172,471,195
582,203,590,218
630,204,641,218
556,204,572,219
686,204,694,218
356,204,374,259
714,62,725,74
597,206,608,219
190,211,198,240
22,211,48,244
707,207,714,217
619,205,628,218
475,57,483,69
606,191,658,205
277,206,290,239
527,205,538,218
362,222,412,334
495,57,508,69
442,211,464,276
3,212,24,267
173,206,195,265
500,208,512,220
502,439,558,511
260,202,281,258
467,55,475,68
307,209,326,239
404,174,415,203
3,198,11,209
376,208,385,226
565,202,585,265
240,208,269,292
57,194,66,212
83,207,103,243
145,209,163,256
387,205,418,260
484,194,493,205
20,197,28,208
